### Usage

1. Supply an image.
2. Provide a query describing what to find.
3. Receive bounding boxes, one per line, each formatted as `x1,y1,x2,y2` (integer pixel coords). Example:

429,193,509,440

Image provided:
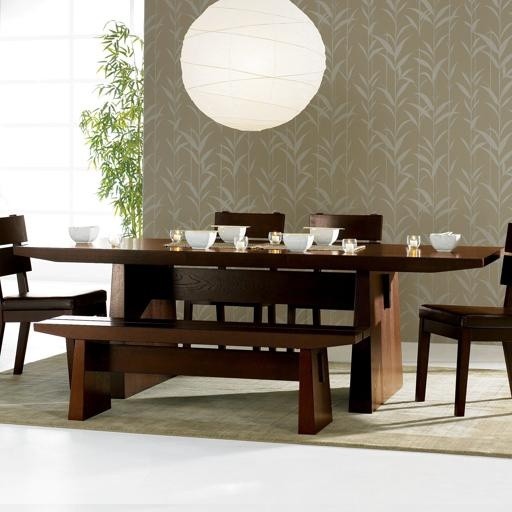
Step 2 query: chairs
267,212,385,351
182,209,288,354
412,222,512,419
0,214,107,394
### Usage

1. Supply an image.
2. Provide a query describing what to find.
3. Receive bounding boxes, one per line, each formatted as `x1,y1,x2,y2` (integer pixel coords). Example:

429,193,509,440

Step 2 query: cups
341,238,357,254
406,234,421,250
234,236,249,251
268,231,282,245
169,229,183,245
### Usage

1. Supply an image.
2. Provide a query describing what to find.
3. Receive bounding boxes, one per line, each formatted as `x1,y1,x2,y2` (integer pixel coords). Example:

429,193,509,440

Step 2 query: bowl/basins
309,227,339,247
430,233,458,253
283,233,313,252
185,230,217,251
218,225,248,243
68,226,99,244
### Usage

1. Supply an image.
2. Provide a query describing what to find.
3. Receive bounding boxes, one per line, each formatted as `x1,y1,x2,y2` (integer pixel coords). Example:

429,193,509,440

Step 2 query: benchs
30,313,372,435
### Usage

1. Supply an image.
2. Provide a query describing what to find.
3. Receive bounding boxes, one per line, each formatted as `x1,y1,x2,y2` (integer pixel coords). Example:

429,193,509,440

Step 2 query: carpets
1,348,512,460
